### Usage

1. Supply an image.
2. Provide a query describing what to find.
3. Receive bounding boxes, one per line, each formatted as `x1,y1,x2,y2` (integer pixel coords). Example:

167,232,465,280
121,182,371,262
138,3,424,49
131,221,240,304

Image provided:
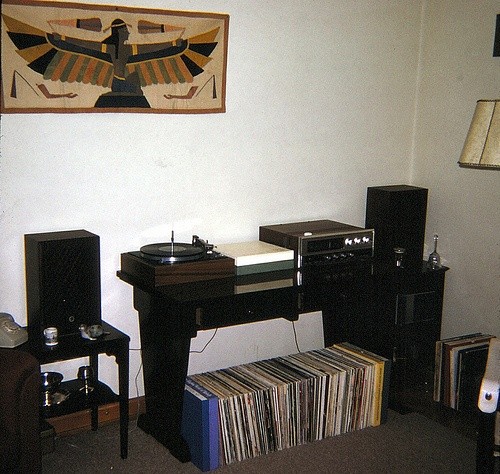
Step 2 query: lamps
457,99,500,169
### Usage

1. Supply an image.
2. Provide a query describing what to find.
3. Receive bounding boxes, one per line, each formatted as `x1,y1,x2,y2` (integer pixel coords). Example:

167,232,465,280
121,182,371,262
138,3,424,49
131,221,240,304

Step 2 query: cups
44,328,58,346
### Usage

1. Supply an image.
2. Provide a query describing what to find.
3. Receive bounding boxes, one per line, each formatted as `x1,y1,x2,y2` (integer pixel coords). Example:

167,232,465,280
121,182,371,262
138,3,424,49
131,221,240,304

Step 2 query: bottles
428,235,440,270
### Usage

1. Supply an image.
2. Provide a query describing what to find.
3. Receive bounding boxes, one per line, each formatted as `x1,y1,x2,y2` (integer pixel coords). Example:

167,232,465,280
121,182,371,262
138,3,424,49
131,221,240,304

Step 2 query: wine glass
41,372,63,406
78,366,94,394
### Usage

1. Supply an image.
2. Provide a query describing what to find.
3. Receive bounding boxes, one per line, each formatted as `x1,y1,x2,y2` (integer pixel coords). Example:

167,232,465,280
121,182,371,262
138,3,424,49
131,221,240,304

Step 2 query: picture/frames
0,0,231,114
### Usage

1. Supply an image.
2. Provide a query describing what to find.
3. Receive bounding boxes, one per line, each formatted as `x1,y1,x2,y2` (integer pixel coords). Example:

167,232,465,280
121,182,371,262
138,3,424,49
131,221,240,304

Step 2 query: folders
182,375,219,472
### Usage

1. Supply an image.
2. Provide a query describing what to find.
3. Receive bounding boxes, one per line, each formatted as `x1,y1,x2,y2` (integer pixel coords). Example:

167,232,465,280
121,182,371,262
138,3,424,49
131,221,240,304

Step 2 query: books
435,332,496,413
181,342,392,472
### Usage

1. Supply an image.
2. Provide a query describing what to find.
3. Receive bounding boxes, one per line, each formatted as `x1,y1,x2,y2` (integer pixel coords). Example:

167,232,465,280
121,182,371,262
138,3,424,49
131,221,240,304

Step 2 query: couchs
0,349,40,474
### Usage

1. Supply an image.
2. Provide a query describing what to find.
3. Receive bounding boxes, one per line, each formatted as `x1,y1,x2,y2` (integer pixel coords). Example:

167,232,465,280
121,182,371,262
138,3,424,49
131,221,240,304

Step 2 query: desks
16,319,131,459
116,259,450,464
